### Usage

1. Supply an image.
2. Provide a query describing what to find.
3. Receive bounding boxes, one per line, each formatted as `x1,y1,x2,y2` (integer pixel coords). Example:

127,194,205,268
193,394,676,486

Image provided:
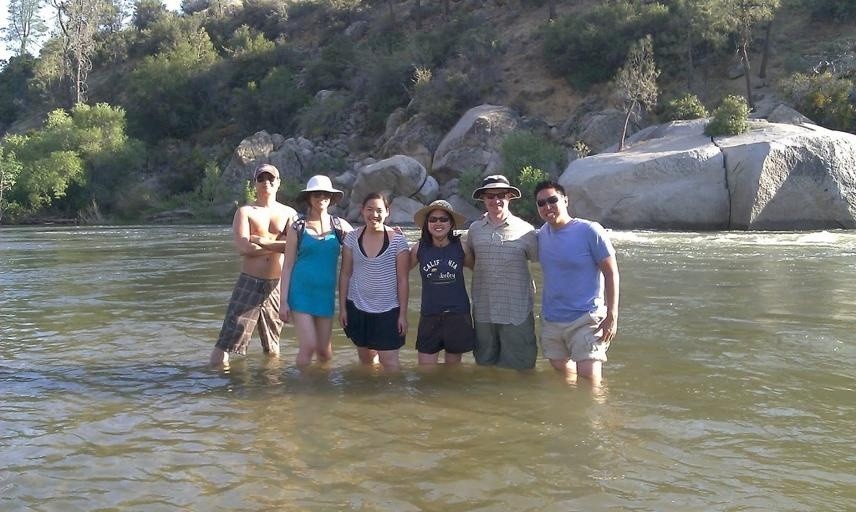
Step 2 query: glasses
536,195,559,207
311,191,330,198
257,175,274,182
483,192,507,199
428,216,448,223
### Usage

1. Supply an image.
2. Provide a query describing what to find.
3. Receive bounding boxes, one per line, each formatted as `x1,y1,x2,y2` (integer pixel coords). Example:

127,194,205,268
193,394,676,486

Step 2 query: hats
295,175,344,207
414,200,464,229
472,174,521,201
253,164,279,177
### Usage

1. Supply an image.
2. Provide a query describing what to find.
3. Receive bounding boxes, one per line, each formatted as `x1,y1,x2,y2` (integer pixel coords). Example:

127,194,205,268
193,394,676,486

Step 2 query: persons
534,180,619,380
392,200,473,365
466,175,539,369
339,191,410,366
209,163,299,366
279,175,344,363
